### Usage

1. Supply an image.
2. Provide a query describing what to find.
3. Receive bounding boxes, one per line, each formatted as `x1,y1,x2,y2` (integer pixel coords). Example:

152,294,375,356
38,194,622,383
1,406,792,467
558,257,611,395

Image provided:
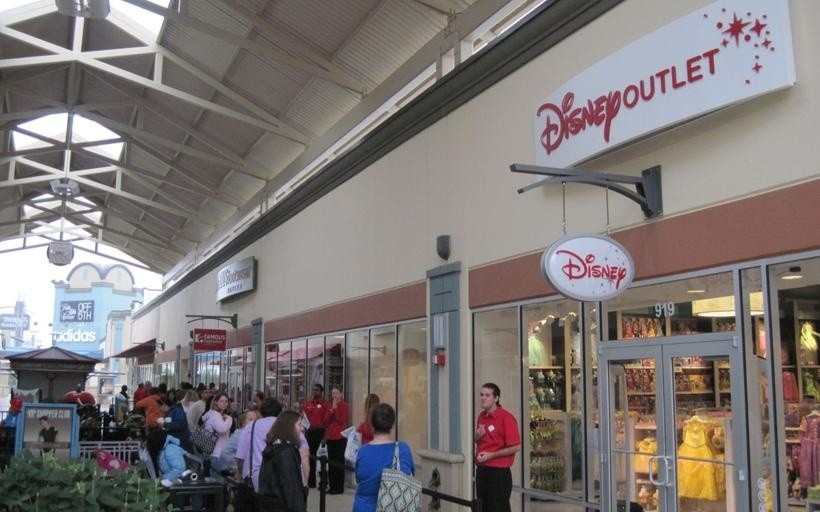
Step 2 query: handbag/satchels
188,410,223,456
372,440,425,512
231,474,267,512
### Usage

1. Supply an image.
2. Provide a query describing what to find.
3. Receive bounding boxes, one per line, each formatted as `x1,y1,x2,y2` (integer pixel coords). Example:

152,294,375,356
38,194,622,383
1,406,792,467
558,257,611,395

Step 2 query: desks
165,477,225,512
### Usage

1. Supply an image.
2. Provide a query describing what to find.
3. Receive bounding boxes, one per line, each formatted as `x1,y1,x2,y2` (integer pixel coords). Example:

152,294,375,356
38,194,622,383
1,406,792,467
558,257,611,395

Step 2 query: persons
39,416,58,456
474,382,521,512
120,380,415,512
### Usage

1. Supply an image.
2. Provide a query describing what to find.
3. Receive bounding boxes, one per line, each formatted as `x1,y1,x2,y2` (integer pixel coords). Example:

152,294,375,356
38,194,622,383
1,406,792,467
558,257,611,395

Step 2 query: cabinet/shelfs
528,409,570,502
528,366,600,426
778,300,820,403
621,314,718,419
616,413,820,511
713,315,769,412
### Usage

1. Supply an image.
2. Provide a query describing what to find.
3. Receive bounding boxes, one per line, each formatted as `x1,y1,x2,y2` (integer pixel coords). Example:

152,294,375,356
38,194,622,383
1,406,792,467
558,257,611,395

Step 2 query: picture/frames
19,402,77,461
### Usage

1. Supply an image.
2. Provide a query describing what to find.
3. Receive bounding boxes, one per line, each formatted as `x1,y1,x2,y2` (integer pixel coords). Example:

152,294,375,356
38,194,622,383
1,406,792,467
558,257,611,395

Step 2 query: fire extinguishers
434,348,447,366
